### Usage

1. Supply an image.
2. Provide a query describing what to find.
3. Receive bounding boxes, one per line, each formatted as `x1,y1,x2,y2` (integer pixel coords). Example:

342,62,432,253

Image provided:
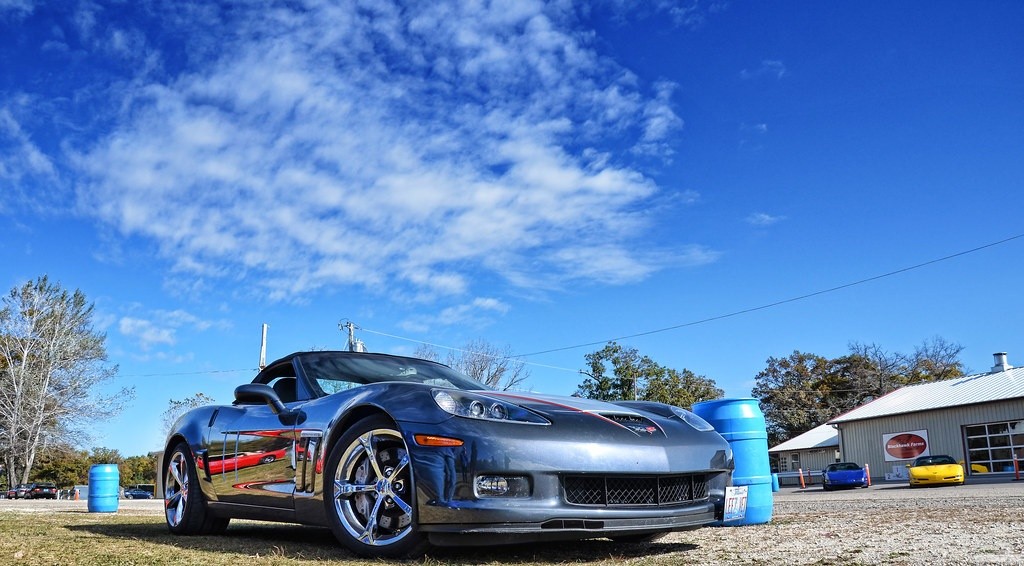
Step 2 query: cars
7,484,28,500
60,490,70,495
295,443,312,462
0,483,7,495
197,449,286,476
232,480,313,497
24,483,58,500
125,489,154,499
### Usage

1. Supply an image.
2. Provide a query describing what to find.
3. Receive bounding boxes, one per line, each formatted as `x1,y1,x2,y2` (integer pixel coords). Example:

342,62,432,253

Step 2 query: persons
67,490,70,500
59,490,63,499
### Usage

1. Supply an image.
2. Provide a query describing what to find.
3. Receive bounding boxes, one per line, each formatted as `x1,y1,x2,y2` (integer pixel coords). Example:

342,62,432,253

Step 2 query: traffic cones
74,491,79,501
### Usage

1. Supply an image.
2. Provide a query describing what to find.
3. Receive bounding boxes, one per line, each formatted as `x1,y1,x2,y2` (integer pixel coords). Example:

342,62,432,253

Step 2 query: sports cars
906,455,965,489
822,462,869,490
971,464,988,473
161,351,748,563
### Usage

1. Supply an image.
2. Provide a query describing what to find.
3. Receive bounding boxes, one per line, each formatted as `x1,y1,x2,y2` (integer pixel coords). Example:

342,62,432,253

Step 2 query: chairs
272,377,298,402
829,466,855,471
919,460,949,466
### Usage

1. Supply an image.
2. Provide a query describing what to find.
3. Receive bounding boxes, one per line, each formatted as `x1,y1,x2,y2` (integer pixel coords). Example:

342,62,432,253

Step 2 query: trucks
129,484,154,494
